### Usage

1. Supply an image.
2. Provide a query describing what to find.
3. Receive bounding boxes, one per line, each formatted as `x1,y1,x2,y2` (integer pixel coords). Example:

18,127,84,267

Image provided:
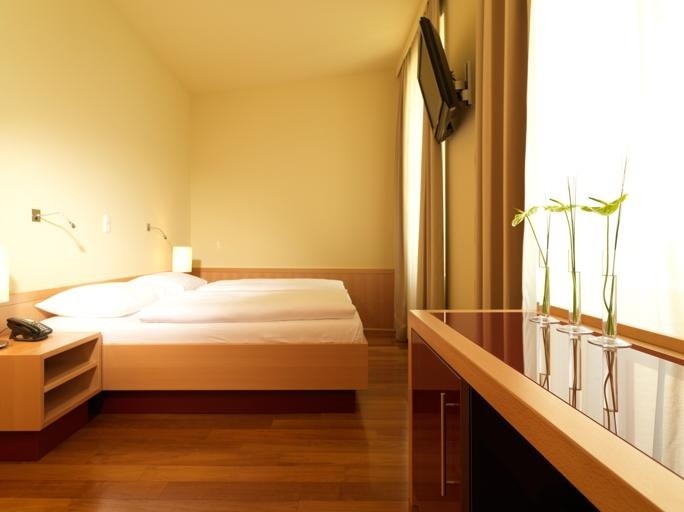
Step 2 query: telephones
7,317,52,341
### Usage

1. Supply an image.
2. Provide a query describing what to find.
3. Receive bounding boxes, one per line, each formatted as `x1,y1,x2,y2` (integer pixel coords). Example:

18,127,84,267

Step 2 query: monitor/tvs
416,16,458,145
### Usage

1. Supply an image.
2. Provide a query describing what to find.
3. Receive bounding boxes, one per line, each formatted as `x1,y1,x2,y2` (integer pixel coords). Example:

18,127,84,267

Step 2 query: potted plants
547,177,593,336
583,149,634,352
509,198,560,326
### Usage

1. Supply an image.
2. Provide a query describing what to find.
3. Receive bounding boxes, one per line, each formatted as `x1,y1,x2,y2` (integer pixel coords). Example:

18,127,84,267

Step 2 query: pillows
35,282,156,319
128,272,207,299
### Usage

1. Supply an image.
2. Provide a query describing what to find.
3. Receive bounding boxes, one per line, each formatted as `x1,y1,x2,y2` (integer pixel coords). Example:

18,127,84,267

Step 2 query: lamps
170,245,193,272
144,222,168,242
30,208,75,231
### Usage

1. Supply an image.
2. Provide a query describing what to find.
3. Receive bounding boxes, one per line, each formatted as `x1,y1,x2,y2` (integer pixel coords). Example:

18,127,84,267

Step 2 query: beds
31,270,371,415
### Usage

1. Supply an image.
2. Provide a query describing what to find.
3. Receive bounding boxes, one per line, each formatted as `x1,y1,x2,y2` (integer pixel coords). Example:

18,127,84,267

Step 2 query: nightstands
0,334,104,462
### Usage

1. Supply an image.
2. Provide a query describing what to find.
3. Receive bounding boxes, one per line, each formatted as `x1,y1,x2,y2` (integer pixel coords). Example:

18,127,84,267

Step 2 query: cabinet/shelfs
399,306,684,512
